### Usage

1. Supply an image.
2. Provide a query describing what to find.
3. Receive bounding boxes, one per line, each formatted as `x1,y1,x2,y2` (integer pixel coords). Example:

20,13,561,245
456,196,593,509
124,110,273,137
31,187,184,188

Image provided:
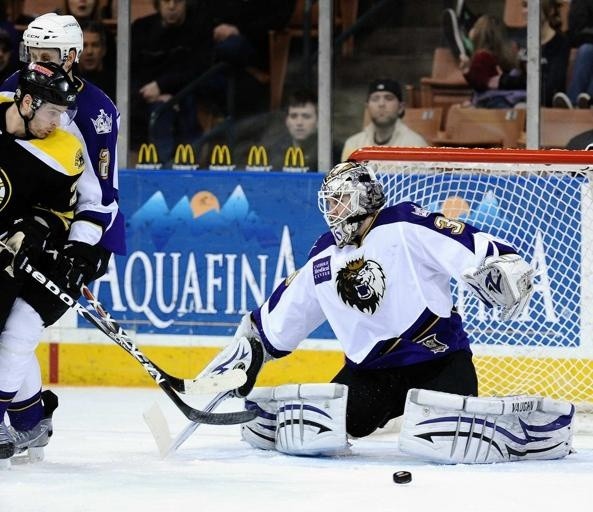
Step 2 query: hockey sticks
78,282,259,424
0,239,248,396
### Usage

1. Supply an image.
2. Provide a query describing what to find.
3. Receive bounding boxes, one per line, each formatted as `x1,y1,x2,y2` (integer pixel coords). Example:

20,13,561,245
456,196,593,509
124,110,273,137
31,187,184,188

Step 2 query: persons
257,95,343,171
552,43,593,108
200,160,577,466
340,78,428,163
0,60,112,460
485,0,572,108
1,0,117,161
1,9,127,281
128,1,297,152
462,15,502,99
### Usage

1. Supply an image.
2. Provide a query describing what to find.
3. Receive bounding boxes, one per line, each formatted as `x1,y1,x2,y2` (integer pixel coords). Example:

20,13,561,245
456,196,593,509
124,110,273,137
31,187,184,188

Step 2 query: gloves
4,215,91,288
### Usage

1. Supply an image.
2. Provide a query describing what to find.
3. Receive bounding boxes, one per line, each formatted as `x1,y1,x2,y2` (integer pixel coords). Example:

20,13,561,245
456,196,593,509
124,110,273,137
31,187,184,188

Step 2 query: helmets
318,161,386,247
14,13,83,126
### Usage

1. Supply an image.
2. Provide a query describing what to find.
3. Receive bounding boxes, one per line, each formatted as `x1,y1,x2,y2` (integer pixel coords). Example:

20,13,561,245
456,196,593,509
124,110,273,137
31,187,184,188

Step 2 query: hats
369,79,401,100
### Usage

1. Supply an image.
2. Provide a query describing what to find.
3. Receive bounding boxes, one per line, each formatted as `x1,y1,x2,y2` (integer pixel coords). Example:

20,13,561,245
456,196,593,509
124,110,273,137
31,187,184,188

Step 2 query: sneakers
0,390,58,459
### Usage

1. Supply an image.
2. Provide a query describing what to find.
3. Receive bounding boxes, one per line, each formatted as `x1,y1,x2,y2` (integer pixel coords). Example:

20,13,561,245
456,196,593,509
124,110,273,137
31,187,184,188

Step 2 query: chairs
14,0,593,146
